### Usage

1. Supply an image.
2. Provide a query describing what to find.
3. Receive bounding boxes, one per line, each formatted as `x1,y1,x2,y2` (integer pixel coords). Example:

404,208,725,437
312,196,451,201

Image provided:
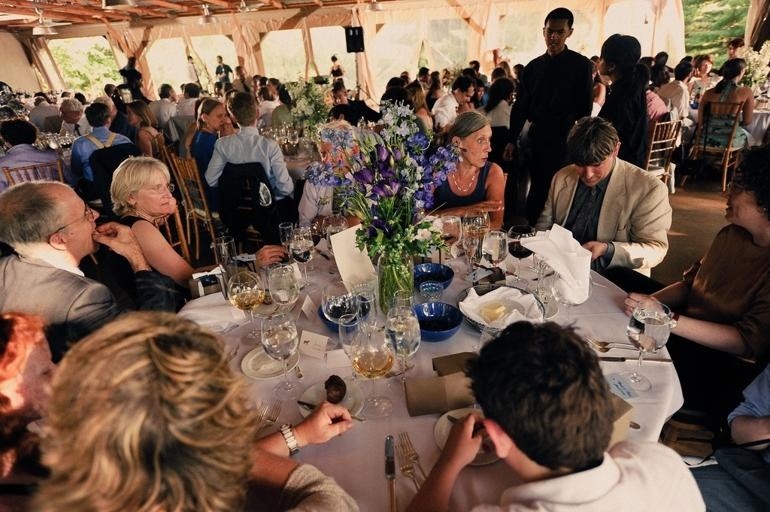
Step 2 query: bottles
694,87,701,106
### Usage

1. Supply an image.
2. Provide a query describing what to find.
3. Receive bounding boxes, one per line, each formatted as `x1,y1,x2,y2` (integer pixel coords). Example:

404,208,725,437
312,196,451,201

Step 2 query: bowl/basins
318,296,370,333
457,284,545,334
413,301,463,342
413,262,454,291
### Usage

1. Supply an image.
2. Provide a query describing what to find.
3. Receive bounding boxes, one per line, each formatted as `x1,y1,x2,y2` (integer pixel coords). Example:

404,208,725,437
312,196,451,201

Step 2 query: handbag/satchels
680,155,711,180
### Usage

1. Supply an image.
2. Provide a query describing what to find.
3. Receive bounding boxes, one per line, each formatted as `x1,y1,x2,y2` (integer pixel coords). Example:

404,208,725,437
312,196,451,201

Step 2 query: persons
500,8,595,232
392,317,707,512
688,361,769,512
214,55,234,83
1,178,180,364
2,66,308,227
0,310,362,512
326,104,354,125
323,82,381,127
105,153,288,295
587,33,770,193
328,55,344,80
295,120,367,247
377,59,525,130
118,58,142,90
619,144,769,396
530,116,673,279
418,110,508,234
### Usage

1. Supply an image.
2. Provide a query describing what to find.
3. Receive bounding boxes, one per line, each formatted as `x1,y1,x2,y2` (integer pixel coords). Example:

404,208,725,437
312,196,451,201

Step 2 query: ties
240,80,250,92
331,178,347,216
571,185,597,244
74,123,81,136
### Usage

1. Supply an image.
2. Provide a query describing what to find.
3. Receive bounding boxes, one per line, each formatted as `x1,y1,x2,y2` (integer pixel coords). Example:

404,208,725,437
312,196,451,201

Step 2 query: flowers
734,40,770,91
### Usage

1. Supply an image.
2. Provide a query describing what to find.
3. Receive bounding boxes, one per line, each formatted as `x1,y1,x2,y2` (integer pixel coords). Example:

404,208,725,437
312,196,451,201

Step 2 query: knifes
385,435,397,511
297,400,364,422
598,356,672,364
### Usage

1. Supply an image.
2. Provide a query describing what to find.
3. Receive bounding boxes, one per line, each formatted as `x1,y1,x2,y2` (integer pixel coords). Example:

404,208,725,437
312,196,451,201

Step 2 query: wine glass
440,206,553,300
268,264,300,313
260,312,304,399
622,301,671,392
756,80,768,99
258,118,315,163
339,282,444,423
35,131,75,157
277,212,349,292
227,272,265,345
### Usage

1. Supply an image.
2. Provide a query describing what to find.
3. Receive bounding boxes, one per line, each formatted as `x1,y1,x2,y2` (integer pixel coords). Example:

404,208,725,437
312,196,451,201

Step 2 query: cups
551,271,592,308
213,237,238,285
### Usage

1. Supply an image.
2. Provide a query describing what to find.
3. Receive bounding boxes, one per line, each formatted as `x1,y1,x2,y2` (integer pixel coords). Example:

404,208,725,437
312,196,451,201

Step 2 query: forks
397,432,428,491
255,400,283,436
585,335,659,353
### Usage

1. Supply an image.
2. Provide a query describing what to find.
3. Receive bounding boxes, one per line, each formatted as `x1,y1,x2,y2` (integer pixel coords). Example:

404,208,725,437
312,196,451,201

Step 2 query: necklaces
451,168,477,195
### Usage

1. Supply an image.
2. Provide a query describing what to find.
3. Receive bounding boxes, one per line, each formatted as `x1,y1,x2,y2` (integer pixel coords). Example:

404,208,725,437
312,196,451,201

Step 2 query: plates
535,300,560,319
434,408,500,466
298,381,364,420
242,344,300,380
252,291,295,318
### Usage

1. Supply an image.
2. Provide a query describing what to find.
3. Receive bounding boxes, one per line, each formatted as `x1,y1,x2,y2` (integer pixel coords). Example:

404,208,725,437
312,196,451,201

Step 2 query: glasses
50,202,95,237
731,177,758,196
138,182,175,196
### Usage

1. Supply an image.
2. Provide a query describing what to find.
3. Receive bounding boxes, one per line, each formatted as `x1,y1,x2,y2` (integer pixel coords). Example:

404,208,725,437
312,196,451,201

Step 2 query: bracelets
669,312,680,329
279,423,302,458
